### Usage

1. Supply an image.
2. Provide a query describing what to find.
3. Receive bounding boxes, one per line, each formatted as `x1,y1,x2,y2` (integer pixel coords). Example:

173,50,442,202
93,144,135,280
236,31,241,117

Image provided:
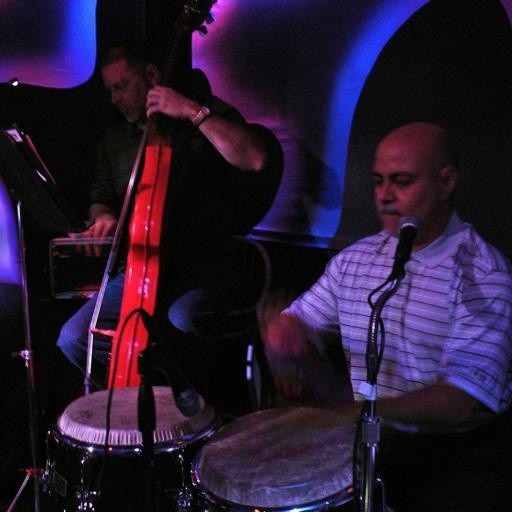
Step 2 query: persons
54,43,271,392
205,120,511,512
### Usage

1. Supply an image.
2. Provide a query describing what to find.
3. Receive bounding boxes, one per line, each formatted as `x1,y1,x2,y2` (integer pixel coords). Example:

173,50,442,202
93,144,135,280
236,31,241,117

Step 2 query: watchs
192,105,210,126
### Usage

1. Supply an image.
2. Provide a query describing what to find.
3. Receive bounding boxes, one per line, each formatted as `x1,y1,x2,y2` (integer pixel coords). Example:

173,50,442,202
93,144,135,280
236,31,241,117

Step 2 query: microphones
140,303,201,420
390,217,420,290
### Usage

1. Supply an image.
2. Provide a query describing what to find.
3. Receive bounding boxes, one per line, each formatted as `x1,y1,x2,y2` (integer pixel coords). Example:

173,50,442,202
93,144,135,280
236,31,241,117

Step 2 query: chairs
204,237,272,414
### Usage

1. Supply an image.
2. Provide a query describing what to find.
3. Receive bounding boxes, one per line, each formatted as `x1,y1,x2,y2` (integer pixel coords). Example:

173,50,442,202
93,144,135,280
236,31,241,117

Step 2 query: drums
40,386,221,511
176,406,388,511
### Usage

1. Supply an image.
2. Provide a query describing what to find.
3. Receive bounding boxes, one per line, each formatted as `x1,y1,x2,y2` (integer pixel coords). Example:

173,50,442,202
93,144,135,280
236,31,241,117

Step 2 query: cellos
83,1,217,389
